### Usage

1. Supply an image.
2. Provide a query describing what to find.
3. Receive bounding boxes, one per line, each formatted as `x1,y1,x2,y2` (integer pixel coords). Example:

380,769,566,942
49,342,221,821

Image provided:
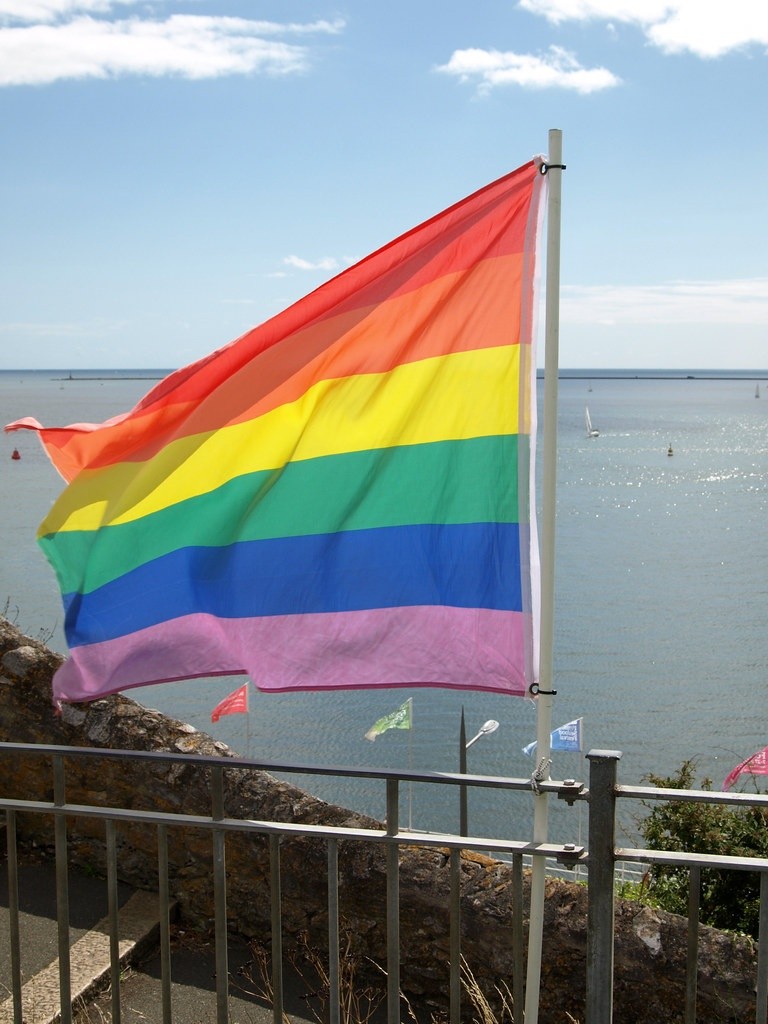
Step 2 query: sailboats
584,405,600,438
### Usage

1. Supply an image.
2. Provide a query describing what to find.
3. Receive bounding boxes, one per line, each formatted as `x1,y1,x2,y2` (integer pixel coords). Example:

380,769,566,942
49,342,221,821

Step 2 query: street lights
457,703,500,839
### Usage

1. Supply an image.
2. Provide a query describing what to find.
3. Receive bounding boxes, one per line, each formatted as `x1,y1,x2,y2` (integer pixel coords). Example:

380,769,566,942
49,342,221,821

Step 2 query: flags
723,744,768,793
365,699,410,742
211,683,248,723
522,718,582,757
5,150,543,714
